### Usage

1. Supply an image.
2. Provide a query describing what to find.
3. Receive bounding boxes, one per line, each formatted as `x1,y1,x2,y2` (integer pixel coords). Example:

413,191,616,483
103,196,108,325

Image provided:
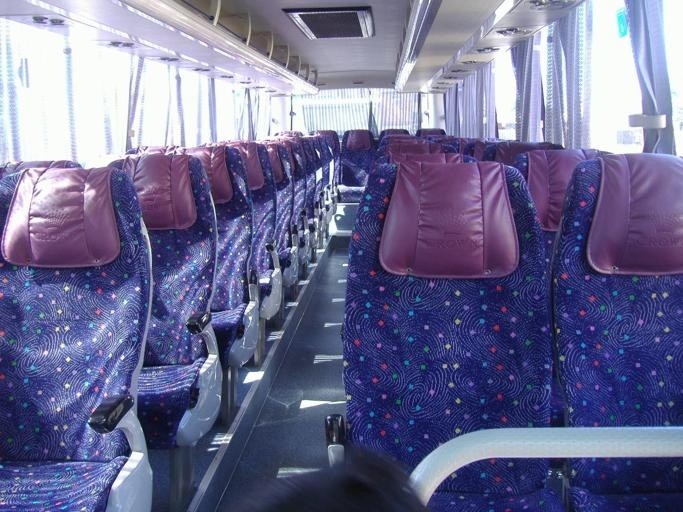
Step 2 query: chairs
379,129,409,146
374,143,458,157
463,138,495,160
268,139,308,301
373,153,480,167
325,163,564,512
426,135,454,143
107,153,223,507
272,140,308,280
338,130,377,202
552,154,683,511
0,169,154,512
262,143,297,331
309,136,332,240
314,136,338,214
309,130,340,186
227,142,282,367
0,161,82,176
454,138,475,153
173,145,260,427
481,142,564,165
510,149,616,258
379,139,436,146
285,139,316,262
416,129,446,136
123,146,181,155
301,136,323,249
381,134,419,138
275,131,303,136
373,143,458,161
